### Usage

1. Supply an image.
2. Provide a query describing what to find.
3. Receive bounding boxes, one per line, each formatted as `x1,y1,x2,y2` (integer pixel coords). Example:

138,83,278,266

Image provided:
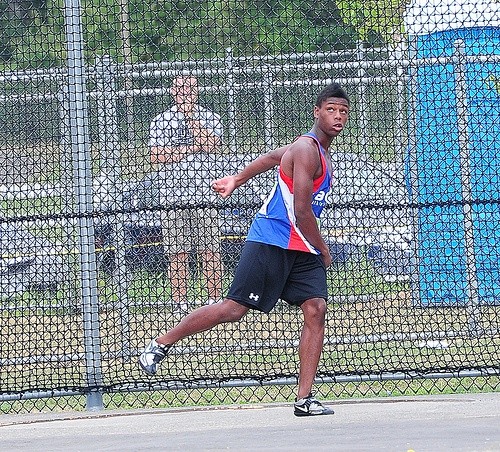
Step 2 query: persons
146,70,229,325
137,80,352,417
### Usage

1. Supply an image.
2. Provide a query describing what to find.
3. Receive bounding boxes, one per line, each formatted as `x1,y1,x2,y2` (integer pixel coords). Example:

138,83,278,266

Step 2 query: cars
93,151,420,284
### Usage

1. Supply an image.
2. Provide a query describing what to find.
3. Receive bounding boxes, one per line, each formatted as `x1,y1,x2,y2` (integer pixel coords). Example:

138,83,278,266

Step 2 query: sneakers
168,307,188,317
294,394,334,416
139,337,169,374
